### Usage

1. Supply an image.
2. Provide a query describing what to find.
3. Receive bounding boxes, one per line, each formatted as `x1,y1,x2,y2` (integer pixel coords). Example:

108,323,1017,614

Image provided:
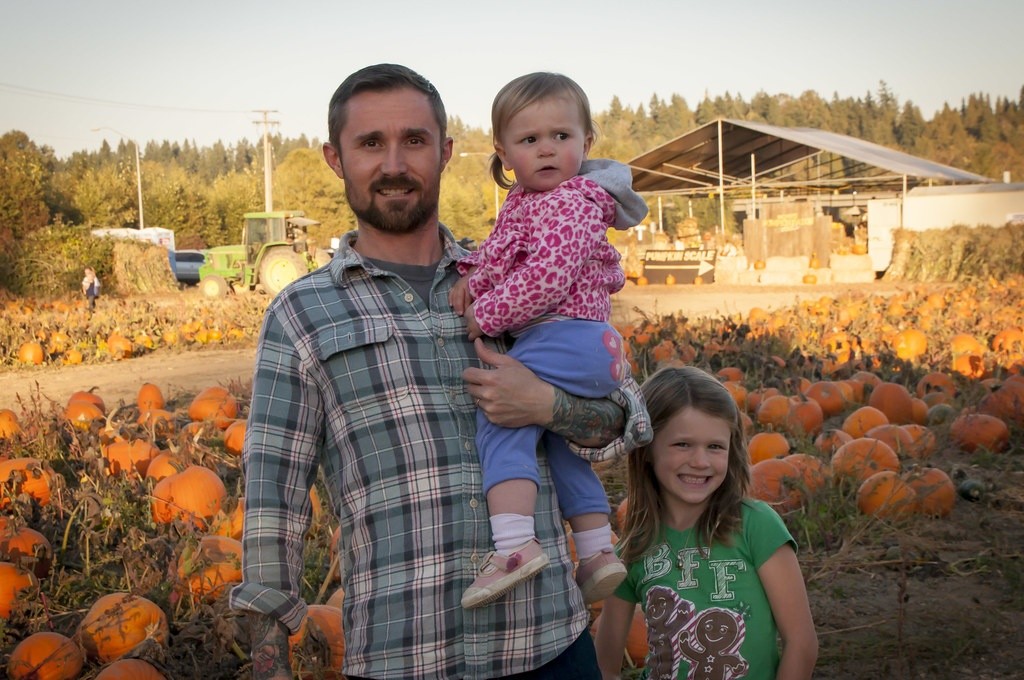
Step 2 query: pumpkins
611,244,1024,522
559,495,653,671
0,318,352,680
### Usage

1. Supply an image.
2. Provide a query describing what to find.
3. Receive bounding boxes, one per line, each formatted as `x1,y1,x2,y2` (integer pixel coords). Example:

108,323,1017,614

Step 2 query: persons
229,64,653,680
594,366,819,680
82,266,100,320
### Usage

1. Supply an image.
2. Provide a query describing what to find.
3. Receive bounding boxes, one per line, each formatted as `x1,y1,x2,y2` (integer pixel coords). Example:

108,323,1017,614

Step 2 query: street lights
91,125,144,230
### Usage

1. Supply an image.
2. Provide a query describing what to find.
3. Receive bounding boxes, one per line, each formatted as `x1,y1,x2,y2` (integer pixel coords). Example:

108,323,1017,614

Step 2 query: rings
476,399,480,407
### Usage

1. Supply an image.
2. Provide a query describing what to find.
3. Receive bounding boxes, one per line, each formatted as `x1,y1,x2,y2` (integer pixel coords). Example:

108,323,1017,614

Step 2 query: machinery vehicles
198,210,332,299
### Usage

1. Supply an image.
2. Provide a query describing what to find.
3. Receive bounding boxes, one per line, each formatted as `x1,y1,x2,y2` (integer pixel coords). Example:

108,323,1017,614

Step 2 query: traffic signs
642,249,716,284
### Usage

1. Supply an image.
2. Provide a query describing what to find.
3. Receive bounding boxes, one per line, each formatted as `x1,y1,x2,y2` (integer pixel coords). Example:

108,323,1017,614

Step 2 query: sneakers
462,537,551,608
575,547,628,605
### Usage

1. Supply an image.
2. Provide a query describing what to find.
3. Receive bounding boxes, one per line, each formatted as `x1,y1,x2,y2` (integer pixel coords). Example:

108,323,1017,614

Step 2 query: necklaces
663,519,696,567
